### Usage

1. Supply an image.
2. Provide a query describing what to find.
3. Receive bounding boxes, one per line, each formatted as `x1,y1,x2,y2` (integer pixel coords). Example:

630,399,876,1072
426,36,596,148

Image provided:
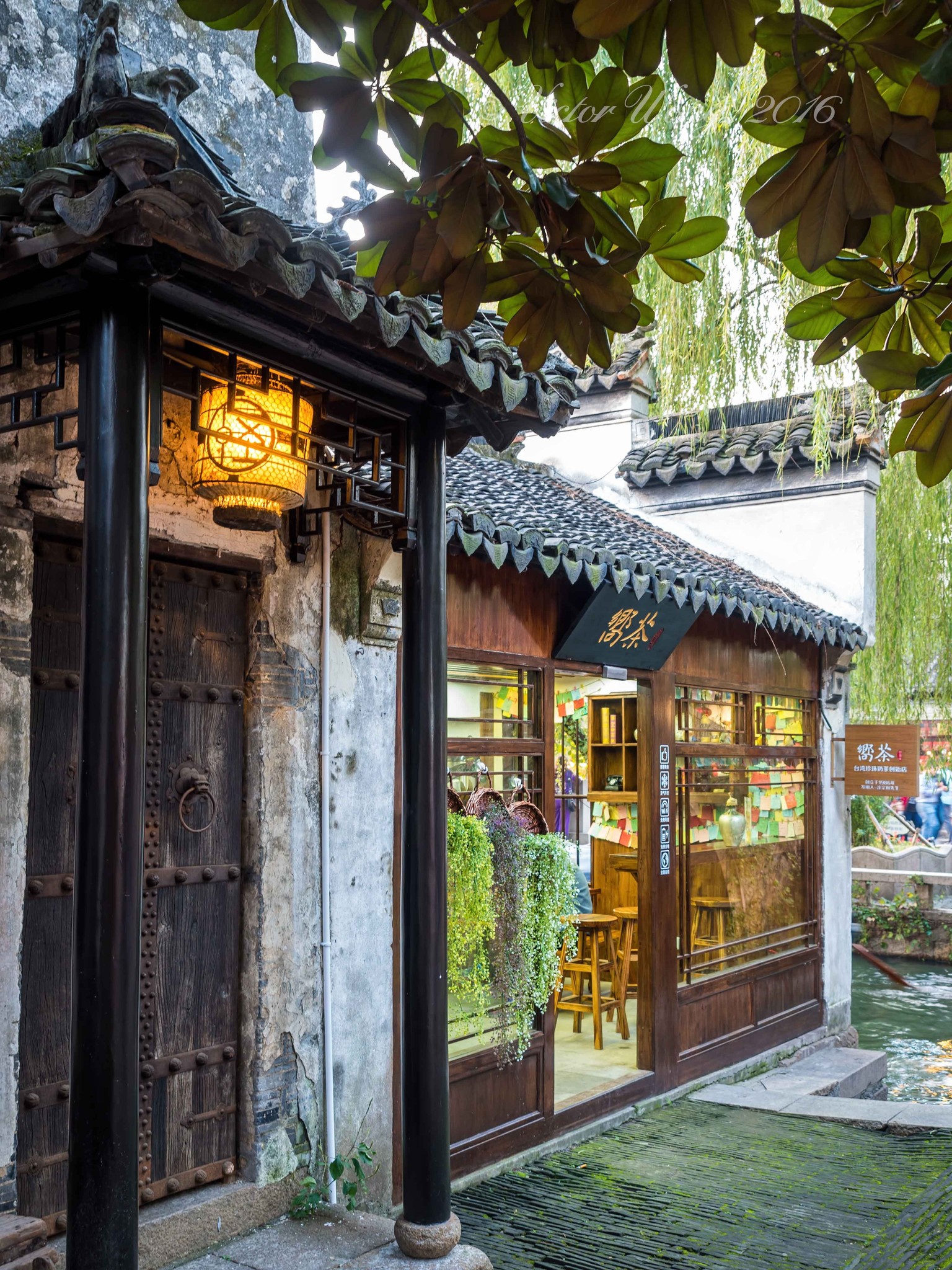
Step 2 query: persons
894,751,952,844
555,756,581,840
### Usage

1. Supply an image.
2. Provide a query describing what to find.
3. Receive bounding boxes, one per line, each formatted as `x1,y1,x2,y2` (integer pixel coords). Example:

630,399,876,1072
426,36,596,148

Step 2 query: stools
551,907,638,1050
682,897,744,979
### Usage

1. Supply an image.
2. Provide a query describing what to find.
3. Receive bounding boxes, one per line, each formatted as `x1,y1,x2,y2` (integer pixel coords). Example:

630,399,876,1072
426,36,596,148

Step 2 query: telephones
605,775,623,791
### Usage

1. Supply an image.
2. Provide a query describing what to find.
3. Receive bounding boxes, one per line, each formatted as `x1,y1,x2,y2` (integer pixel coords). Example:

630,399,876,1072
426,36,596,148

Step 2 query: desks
609,839,803,884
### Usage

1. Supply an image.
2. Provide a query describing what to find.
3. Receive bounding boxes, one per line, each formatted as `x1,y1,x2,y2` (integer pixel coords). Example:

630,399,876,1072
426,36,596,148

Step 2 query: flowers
446,801,583,1069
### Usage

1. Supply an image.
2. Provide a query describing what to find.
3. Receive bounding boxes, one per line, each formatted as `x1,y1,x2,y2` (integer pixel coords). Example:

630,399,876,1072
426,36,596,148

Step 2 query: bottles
718,805,745,847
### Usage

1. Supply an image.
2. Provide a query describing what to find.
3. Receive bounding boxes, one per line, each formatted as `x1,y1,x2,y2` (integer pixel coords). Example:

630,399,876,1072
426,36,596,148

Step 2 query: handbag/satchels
568,792,581,812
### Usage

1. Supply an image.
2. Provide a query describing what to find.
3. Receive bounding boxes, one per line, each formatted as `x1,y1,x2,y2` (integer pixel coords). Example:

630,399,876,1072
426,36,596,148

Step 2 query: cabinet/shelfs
585,693,638,983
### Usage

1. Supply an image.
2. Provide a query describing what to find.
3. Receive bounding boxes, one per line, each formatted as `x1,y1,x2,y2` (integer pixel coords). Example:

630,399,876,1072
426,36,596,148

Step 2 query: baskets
467,770,512,821
446,769,469,820
508,786,549,837
